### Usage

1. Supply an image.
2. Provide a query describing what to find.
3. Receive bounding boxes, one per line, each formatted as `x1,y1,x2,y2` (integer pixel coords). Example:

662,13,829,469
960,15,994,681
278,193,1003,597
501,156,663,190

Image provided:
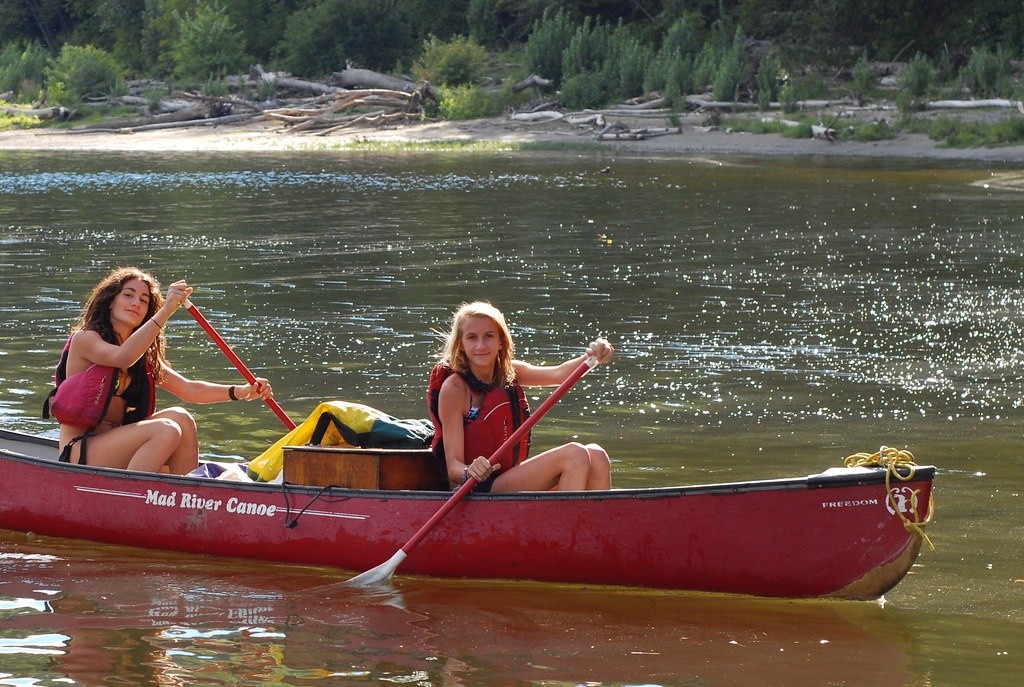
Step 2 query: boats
0,427,934,601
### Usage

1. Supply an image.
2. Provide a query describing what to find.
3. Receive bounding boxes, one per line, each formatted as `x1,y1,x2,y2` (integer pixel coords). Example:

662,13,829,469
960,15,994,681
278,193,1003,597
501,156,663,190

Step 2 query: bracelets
462,465,469,483
228,385,237,401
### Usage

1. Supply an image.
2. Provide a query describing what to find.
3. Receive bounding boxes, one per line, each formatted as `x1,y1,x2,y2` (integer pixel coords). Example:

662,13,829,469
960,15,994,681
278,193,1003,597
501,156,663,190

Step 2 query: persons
430,298,619,498
44,267,273,479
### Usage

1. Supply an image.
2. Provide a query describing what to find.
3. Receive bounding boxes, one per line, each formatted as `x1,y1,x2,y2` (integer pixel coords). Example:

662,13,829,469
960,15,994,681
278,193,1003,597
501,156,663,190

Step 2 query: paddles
182,297,295,431
347,352,599,588
